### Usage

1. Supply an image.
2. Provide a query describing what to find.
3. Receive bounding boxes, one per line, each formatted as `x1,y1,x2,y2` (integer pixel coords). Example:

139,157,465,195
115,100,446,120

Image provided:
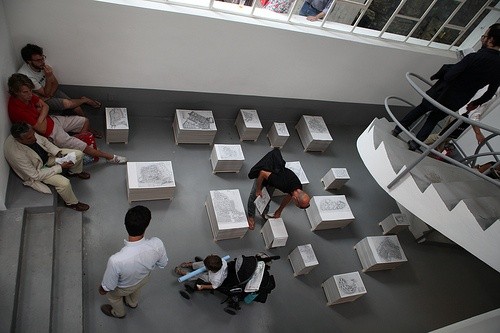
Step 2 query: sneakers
109,153,127,164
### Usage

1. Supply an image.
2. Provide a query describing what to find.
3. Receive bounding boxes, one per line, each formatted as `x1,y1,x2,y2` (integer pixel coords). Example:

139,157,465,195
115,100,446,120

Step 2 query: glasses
30,55,48,63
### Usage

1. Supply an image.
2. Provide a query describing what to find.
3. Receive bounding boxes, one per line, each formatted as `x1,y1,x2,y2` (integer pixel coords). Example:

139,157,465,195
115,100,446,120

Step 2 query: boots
180,261,194,268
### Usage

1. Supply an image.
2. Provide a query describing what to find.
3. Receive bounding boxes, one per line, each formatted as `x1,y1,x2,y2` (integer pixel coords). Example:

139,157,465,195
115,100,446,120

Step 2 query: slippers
247,218,258,230
175,267,186,276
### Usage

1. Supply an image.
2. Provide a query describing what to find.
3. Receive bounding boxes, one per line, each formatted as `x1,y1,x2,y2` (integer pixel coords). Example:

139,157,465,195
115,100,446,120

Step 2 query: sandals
91,129,104,139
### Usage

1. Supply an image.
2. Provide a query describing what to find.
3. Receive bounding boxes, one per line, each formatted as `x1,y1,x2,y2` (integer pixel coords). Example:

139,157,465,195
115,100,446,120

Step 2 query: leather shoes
74,172,93,180
100,303,126,319
391,128,399,136
69,202,91,212
123,296,138,309
407,138,416,152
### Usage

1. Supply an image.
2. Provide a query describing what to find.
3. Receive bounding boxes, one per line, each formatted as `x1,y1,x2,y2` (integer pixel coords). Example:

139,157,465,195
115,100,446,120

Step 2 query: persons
248,168,310,230
99,205,168,319
176,255,228,291
392,23,500,152
299,0,336,22
4,44,127,211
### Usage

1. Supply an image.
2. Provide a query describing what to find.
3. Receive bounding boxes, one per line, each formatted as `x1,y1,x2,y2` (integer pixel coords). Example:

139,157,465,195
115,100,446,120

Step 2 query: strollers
179,252,280,315
